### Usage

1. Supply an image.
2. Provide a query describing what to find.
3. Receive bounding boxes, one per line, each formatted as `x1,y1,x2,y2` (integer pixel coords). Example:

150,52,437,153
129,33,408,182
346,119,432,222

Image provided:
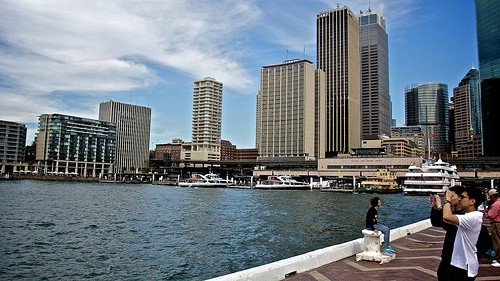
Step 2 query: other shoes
491,262,500,267
387,245,396,252
492,260,497,262
383,247,394,256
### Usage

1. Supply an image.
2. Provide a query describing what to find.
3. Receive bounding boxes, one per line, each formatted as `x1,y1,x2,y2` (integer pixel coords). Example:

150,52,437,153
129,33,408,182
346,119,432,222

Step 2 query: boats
402,154,462,197
178,172,228,188
254,175,311,190
355,167,403,194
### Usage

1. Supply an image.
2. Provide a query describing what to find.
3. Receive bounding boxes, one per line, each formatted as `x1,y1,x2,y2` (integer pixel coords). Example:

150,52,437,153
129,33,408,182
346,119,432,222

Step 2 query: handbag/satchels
475,225,497,259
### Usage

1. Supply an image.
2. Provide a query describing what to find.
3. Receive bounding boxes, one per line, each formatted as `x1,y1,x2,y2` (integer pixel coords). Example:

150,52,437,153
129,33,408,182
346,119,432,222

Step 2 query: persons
481,188,500,267
365,197,395,256
429,185,483,281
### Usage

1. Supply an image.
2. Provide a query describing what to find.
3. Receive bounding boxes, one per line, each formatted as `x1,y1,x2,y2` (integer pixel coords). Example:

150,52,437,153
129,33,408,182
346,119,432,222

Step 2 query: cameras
434,194,438,201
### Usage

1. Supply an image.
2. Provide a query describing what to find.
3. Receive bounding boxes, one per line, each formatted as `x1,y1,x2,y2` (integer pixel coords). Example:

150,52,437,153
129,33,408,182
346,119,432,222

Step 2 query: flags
429,132,436,138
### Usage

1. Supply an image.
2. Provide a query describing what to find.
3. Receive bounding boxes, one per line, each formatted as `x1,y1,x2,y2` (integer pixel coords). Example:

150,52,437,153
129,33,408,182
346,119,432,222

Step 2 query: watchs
444,200,452,204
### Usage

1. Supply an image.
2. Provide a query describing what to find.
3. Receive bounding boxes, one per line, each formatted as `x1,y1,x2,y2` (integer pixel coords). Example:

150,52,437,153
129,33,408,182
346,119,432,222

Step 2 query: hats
488,188,498,198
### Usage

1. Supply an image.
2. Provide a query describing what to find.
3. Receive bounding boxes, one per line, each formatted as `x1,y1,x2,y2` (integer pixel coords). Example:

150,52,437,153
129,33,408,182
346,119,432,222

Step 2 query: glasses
460,195,470,199
